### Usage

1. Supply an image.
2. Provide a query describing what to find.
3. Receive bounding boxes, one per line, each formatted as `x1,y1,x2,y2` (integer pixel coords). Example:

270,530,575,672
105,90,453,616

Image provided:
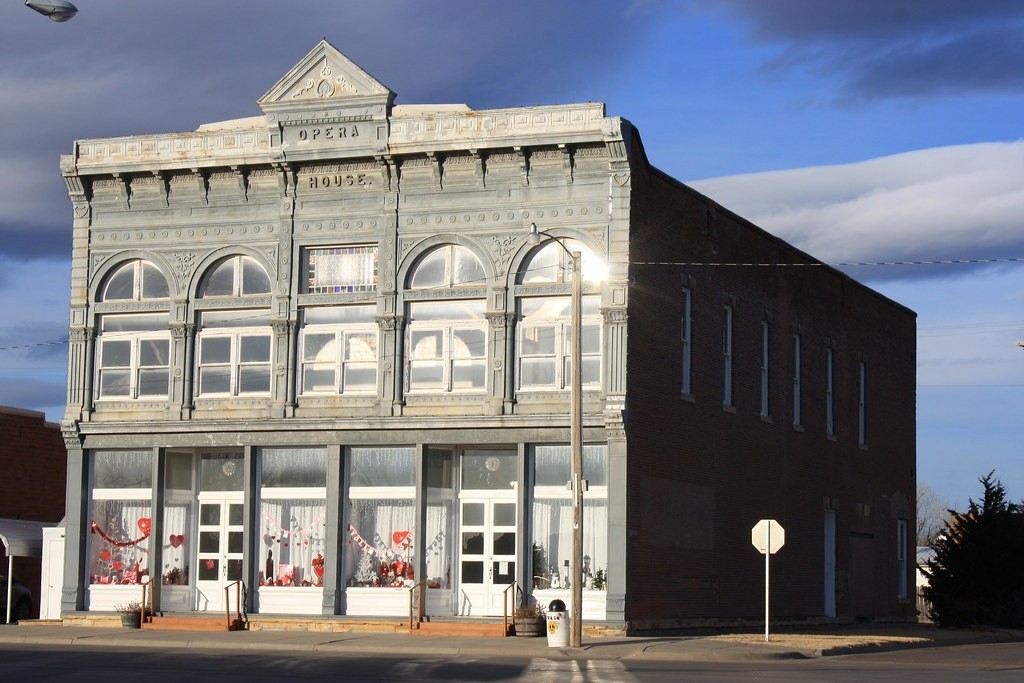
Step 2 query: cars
0,575,33,623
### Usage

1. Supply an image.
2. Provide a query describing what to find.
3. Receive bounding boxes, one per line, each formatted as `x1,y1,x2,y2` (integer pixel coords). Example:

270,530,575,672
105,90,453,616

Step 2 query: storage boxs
90,570,143,583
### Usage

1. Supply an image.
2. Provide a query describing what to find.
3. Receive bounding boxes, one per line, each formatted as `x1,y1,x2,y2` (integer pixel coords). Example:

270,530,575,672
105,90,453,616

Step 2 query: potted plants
119,601,142,631
514,603,545,637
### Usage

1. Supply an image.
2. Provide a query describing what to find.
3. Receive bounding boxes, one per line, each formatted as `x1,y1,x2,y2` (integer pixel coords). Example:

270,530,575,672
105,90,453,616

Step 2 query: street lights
528,222,584,649
